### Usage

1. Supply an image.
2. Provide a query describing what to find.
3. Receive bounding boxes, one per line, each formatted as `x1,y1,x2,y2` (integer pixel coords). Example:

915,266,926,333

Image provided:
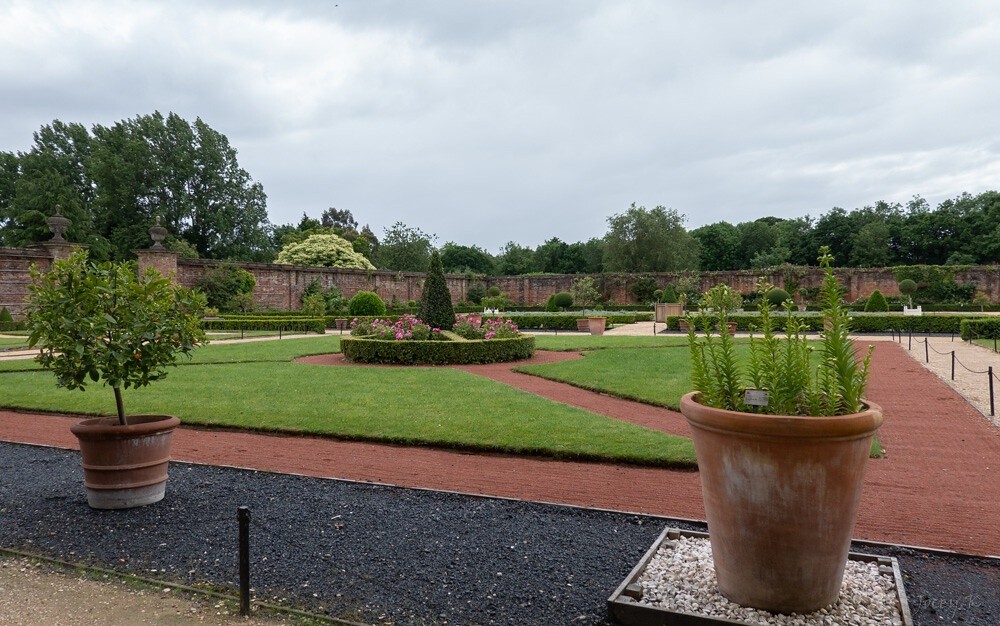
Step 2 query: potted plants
677,294,693,332
587,304,606,334
797,288,809,311
204,307,221,319
705,284,741,337
27,250,211,508
572,275,602,333
330,298,348,330
678,246,884,611
